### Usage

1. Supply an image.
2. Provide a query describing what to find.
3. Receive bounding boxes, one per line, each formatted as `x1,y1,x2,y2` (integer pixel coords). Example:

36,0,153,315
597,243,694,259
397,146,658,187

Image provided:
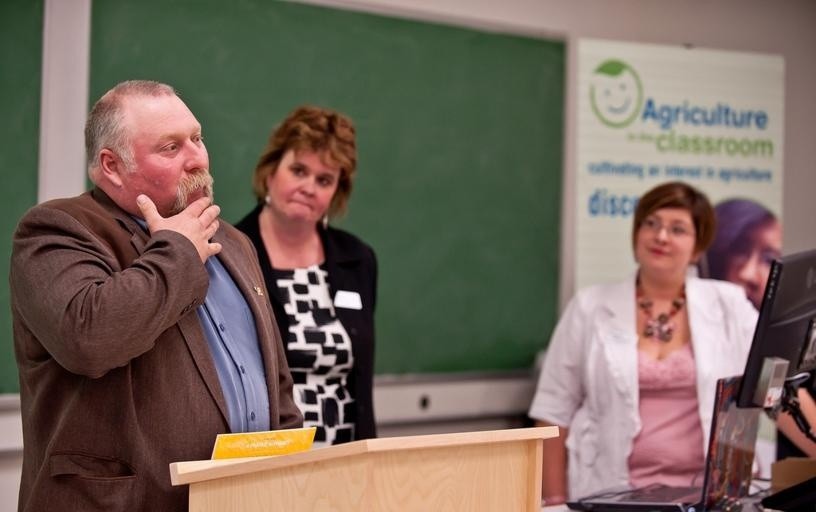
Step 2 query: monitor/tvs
737,249,816,409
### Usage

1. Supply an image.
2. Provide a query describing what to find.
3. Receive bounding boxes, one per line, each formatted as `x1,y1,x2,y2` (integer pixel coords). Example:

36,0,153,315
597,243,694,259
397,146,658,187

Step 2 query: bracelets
542,495,567,504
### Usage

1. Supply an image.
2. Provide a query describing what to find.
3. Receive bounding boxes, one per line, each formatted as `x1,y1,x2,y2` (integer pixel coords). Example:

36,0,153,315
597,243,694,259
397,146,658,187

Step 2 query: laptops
566,376,761,512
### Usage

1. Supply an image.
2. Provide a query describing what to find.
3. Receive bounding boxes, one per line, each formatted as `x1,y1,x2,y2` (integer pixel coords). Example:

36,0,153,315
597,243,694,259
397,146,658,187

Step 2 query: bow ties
645,320,676,342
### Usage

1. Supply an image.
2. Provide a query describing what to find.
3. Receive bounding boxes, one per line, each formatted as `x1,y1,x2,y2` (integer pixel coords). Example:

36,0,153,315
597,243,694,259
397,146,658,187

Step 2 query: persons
528,182,816,512
232,106,378,448
699,199,782,311
8,79,303,510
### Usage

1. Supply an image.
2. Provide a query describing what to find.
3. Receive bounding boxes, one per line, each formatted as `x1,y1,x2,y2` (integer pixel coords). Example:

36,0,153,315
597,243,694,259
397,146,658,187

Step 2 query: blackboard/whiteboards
0,0,576,411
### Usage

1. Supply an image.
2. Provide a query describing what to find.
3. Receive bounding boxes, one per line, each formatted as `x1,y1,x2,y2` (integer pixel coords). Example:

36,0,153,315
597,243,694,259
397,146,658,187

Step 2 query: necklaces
637,274,685,343
